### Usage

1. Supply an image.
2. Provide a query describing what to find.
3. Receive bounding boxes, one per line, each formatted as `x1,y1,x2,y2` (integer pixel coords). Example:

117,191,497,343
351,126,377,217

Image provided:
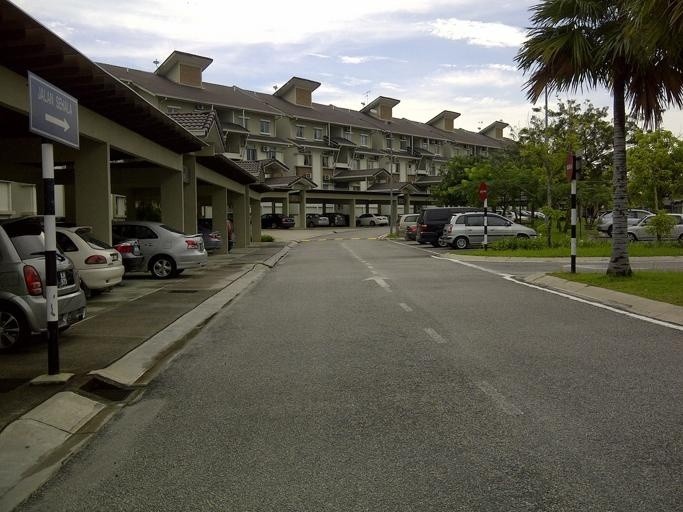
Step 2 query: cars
0,206,236,353
250,209,390,229
394,202,683,248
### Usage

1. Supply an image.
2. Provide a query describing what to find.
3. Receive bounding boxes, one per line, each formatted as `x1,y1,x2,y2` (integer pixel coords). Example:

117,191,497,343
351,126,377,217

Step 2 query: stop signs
477,182,487,201
564,150,574,182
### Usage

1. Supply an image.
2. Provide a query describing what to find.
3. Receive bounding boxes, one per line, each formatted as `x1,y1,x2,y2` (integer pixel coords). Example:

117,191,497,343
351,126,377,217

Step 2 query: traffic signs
26,67,81,152
